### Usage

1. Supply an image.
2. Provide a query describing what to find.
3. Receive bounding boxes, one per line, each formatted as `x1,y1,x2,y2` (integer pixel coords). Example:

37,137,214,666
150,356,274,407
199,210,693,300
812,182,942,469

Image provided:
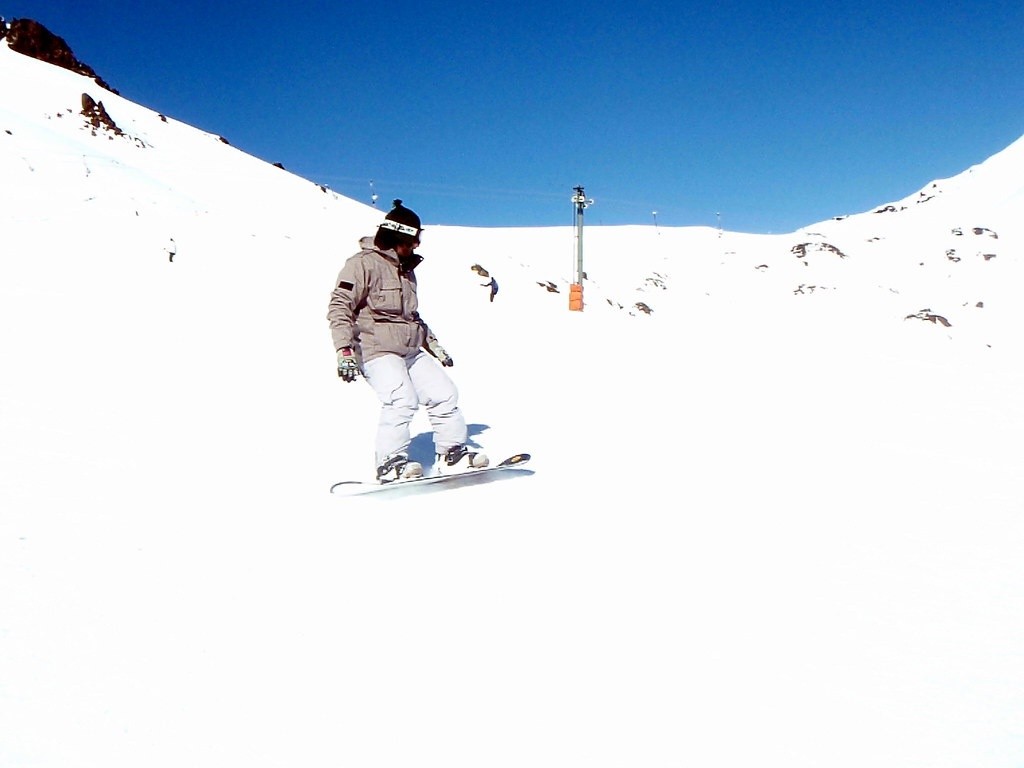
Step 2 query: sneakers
430,445,489,476
376,454,423,485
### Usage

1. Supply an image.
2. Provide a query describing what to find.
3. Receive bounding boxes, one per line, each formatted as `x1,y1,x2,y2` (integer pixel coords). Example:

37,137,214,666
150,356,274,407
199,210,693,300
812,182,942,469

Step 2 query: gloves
337,348,361,383
422,339,453,368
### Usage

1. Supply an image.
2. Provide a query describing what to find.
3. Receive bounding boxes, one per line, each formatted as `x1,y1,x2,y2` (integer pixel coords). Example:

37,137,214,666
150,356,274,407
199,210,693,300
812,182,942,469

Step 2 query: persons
480,277,498,302
164,239,175,262
326,200,489,485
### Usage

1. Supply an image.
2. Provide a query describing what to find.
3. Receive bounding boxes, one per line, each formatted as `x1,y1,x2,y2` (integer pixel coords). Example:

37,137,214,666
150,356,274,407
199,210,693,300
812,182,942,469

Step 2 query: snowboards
330,447,532,498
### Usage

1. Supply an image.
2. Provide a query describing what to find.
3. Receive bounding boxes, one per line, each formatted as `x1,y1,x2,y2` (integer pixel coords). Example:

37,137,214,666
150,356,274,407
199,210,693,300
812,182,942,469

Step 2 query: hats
374,199,425,251
